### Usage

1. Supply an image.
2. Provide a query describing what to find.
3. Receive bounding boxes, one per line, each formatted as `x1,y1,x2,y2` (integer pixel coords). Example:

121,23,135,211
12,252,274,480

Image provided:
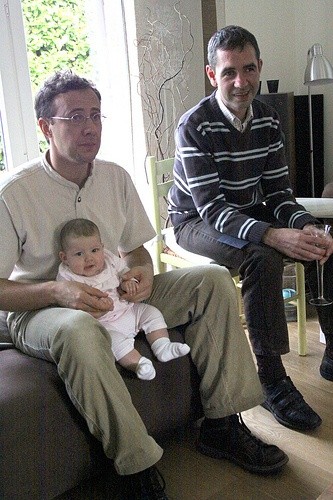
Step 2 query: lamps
303,44,333,198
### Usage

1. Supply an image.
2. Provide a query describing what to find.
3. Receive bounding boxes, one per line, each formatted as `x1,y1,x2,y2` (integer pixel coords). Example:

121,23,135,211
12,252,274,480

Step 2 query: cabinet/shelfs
254,91,324,199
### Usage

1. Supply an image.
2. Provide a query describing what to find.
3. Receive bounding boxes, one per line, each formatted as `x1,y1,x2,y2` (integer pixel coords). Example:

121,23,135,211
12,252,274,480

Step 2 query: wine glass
309,224,333,306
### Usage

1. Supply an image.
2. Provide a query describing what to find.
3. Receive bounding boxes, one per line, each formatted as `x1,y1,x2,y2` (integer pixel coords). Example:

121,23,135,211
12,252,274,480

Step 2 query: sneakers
260,376,322,430
320,351,333,382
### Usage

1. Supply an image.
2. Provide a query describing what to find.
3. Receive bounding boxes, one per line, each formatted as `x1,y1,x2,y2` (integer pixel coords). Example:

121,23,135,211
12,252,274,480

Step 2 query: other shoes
120,466,168,500
197,412,289,473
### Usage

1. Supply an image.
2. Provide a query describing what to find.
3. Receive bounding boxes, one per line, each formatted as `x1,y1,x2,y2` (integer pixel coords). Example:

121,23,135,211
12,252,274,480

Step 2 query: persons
57,218,190,380
168,25,333,431
0,70,289,500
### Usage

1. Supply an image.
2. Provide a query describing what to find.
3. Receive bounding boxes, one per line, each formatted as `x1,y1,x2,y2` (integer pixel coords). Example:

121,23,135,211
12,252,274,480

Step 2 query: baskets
282,264,316,315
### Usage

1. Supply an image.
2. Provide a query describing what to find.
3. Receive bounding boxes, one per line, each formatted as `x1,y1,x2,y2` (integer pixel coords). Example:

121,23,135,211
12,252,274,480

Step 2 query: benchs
0,323,204,500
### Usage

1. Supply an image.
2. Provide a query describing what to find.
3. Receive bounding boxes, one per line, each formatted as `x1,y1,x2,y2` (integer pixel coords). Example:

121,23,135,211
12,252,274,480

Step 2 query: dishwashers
257,81,262,95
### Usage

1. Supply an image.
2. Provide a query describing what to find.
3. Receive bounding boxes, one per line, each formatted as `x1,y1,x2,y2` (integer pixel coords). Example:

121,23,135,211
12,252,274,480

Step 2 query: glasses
51,113,107,126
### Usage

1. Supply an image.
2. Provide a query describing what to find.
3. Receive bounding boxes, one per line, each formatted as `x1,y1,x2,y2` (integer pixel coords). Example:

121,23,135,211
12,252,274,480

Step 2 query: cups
267,79,279,93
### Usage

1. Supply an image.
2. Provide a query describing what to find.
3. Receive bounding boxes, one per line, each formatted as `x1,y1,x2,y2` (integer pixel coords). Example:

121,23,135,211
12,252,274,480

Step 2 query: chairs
146,156,308,356
